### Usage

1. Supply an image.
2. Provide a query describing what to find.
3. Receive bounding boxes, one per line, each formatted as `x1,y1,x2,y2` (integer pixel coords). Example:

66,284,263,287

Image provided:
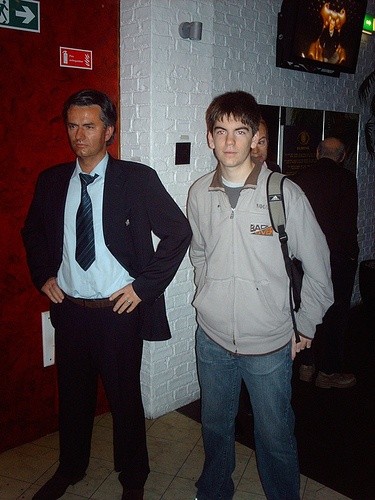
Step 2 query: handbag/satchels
266,171,305,313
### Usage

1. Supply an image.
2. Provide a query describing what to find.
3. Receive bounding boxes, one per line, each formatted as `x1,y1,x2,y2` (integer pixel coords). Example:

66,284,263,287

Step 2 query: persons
251,120,281,173
292,137,359,388
22,89,193,500
187,90,334,500
320,19,340,62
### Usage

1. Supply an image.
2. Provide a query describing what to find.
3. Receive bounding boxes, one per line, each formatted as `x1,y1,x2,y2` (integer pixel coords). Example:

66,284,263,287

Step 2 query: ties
75,173,100,272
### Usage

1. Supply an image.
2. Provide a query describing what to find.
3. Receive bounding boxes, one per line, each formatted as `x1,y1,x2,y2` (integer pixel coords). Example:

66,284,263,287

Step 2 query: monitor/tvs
281,0,367,73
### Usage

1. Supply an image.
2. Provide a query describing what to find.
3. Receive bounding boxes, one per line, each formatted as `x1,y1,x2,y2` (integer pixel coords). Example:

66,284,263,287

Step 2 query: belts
65,294,123,308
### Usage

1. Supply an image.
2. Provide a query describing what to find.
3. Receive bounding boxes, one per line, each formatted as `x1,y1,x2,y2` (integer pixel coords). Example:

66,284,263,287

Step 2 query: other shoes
31,472,87,500
121,486,145,500
300,364,316,381
316,371,357,388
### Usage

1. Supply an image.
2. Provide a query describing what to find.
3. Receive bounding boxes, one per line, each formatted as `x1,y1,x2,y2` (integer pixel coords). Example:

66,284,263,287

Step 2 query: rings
127,300,130,303
